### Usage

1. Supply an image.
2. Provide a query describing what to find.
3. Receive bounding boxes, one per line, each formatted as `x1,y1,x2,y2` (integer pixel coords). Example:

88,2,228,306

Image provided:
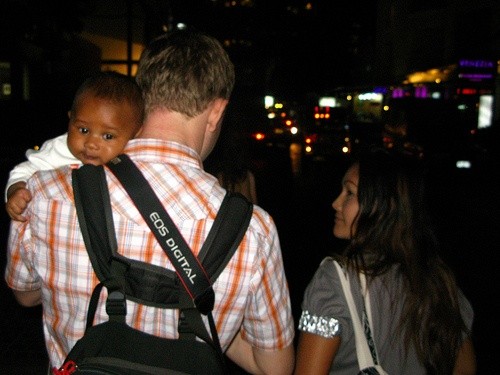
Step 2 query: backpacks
46,163,253,375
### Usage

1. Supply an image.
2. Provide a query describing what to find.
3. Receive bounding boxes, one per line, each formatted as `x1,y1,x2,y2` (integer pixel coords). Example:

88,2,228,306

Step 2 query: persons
4,71,146,225
287,155,479,375
1,30,296,375
